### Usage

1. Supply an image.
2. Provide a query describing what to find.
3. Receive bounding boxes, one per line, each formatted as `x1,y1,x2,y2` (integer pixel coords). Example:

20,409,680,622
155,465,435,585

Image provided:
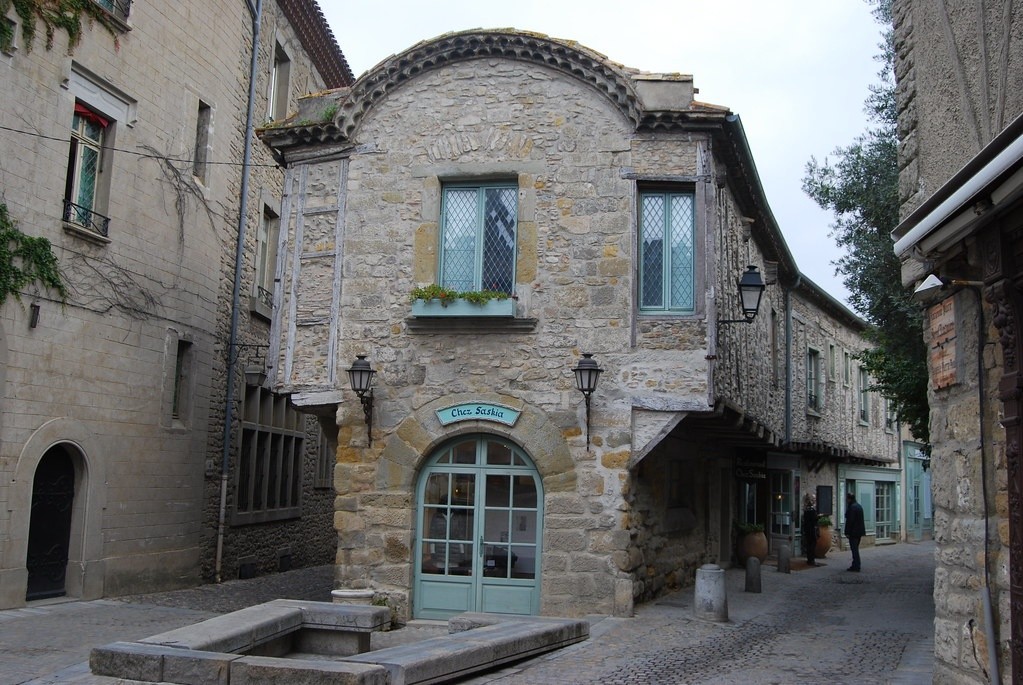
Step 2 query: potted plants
734,521,768,566
814,517,833,557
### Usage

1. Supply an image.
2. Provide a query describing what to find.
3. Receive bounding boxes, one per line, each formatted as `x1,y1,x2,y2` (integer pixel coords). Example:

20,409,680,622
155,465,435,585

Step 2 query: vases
331,590,377,603
411,298,517,318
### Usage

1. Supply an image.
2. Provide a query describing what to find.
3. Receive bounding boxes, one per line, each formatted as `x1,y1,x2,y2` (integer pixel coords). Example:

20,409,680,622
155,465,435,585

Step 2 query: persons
844,491,866,572
801,493,824,566
428,494,464,575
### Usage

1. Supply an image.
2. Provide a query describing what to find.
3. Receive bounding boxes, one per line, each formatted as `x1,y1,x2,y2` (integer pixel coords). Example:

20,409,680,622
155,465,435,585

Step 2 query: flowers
407,284,507,307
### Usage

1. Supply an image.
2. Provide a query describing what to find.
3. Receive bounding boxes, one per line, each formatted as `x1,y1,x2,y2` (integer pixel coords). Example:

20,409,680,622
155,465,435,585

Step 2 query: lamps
225,343,273,387
345,354,377,448
718,265,766,324
571,353,603,451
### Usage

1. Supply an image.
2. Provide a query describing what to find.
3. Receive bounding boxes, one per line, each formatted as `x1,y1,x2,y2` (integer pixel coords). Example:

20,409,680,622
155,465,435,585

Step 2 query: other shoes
847,567,860,572
